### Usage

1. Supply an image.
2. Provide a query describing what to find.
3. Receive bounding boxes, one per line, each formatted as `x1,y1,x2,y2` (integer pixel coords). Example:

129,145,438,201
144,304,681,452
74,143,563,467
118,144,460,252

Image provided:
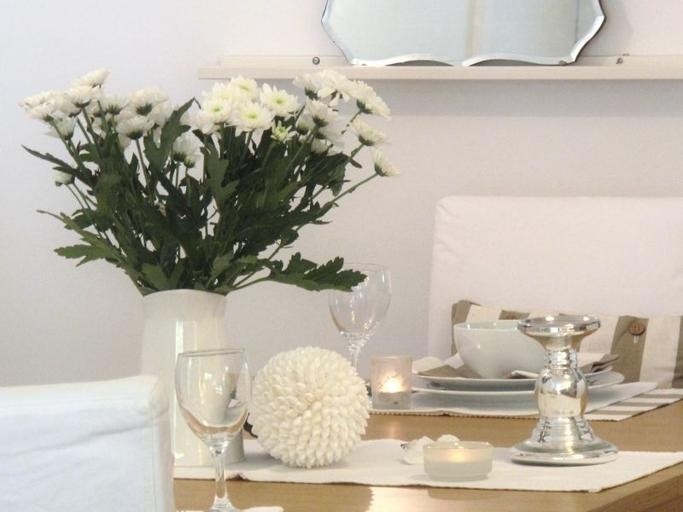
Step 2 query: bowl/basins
453,318,547,379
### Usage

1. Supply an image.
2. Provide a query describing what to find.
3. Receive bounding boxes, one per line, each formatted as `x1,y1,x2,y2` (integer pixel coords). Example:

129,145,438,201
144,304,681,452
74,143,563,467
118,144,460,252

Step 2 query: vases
139,287,246,468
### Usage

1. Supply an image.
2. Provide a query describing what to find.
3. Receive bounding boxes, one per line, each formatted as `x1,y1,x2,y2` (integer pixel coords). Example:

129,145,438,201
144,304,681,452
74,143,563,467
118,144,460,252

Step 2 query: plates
408,371,625,407
409,351,614,391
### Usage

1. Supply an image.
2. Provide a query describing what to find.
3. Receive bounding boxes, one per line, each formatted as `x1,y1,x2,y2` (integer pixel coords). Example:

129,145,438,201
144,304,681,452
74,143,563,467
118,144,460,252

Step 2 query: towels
365,387,683,421
174,438,683,492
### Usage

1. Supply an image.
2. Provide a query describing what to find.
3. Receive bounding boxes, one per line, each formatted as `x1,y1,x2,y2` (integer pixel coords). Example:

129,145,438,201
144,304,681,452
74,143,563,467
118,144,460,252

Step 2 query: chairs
1,374,176,512
427,194,683,388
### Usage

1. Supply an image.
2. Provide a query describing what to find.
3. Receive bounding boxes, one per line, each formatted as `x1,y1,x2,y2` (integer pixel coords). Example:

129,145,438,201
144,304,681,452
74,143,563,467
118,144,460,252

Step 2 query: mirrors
319,0,608,67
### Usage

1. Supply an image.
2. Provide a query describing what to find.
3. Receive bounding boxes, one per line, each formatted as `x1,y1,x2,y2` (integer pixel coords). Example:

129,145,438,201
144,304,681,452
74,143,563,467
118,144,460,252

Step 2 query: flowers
18,66,402,297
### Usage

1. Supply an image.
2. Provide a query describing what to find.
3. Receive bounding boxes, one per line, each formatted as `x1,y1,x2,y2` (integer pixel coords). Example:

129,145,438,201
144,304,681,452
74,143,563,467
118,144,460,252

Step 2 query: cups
369,354,413,411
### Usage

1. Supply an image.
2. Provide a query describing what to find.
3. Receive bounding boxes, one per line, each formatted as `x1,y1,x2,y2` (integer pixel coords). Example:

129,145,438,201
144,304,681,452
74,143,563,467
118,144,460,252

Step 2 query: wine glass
174,346,252,512
327,261,392,369
